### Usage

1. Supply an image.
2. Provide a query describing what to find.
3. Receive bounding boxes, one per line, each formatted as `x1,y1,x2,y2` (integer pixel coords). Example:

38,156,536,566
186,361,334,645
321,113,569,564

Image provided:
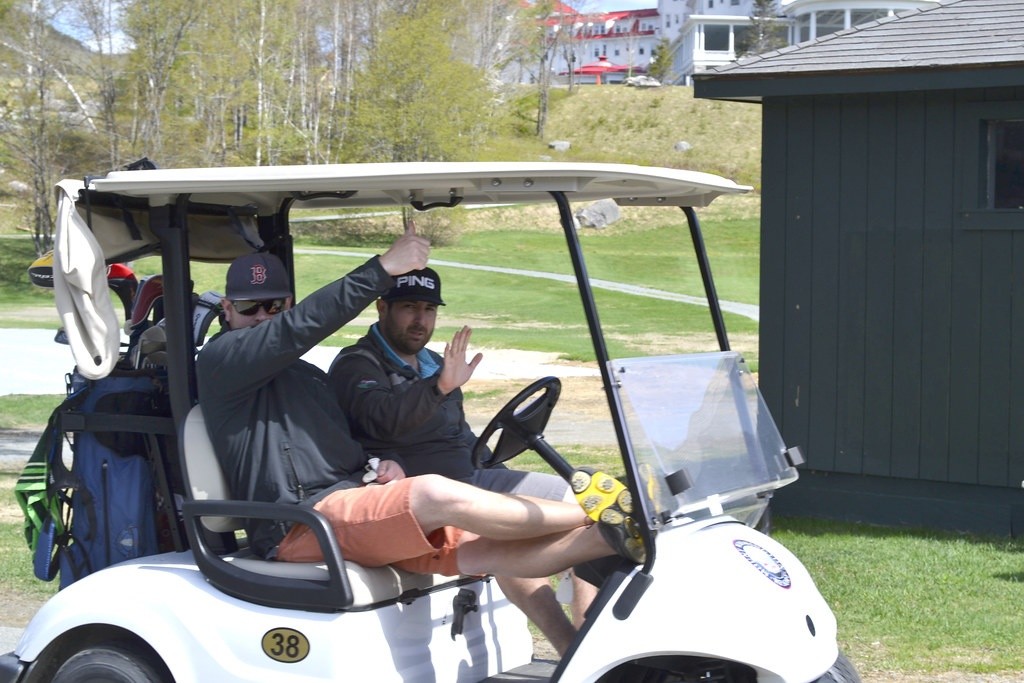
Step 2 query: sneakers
630,462,661,517
569,467,648,564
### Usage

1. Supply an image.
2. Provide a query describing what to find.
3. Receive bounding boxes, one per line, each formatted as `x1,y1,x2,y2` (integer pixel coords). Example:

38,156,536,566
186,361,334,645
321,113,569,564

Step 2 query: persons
197,221,661,578
328,268,600,659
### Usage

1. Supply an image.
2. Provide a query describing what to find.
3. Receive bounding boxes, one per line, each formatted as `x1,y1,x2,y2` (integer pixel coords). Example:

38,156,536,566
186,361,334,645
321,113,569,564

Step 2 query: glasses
231,300,286,316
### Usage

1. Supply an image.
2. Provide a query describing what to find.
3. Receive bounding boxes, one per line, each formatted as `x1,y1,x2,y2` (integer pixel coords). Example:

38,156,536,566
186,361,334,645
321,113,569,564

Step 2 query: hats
225,252,293,301
381,266,445,306
